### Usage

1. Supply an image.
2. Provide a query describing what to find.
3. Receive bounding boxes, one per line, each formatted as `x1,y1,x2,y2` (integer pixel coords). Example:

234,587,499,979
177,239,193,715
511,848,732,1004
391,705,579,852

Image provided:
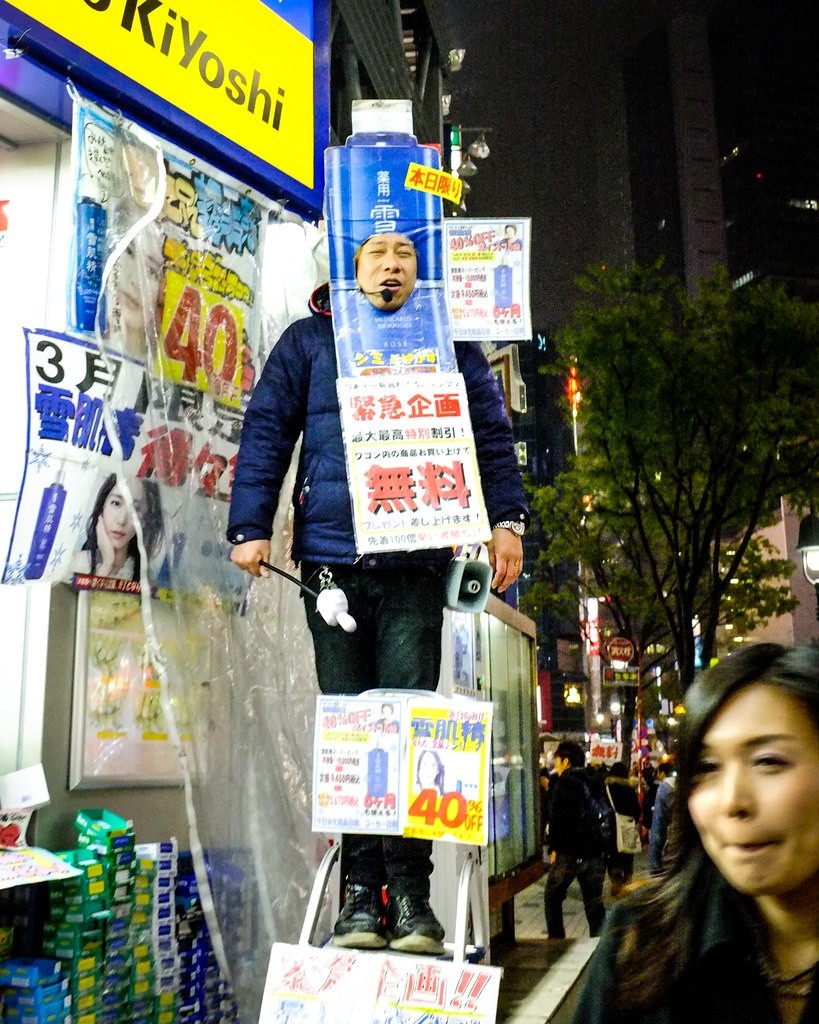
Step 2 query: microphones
362,288,392,303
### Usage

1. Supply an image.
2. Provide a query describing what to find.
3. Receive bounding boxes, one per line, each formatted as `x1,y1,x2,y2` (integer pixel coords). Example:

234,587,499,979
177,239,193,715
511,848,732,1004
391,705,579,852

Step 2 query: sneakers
386,888,445,955
332,875,388,948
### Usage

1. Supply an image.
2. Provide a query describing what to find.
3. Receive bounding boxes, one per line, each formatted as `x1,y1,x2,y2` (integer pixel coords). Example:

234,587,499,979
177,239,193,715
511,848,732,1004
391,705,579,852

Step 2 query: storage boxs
0,806,246,1024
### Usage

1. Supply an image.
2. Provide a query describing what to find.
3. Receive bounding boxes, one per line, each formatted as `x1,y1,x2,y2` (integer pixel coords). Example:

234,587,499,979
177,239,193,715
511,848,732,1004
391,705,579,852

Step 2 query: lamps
457,156,477,177
467,128,490,160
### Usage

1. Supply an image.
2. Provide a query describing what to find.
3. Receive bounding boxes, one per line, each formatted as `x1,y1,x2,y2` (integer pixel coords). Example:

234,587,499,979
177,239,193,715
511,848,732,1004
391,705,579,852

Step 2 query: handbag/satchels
581,783,616,850
615,812,641,855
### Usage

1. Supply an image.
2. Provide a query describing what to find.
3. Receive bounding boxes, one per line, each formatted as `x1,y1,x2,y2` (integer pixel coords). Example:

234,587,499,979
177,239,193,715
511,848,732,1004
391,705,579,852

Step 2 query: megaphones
446,557,494,613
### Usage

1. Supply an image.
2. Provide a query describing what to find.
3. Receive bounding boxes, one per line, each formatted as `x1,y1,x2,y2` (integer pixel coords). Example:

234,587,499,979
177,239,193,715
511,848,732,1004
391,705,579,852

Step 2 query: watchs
494,521,527,537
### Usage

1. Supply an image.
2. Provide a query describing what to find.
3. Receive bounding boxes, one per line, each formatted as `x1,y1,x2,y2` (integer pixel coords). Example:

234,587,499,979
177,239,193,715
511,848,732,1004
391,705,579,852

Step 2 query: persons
573,643,819,1024
77,473,163,580
539,719,677,891
373,703,400,732
499,224,523,251
538,737,617,939
224,229,529,954
